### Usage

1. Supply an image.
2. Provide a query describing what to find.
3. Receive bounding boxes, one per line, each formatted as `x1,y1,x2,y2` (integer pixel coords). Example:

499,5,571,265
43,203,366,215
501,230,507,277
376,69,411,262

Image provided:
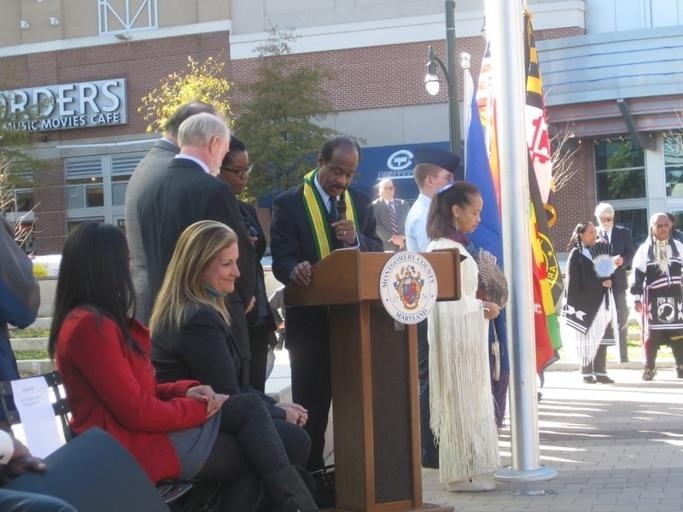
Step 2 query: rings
343,231,346,236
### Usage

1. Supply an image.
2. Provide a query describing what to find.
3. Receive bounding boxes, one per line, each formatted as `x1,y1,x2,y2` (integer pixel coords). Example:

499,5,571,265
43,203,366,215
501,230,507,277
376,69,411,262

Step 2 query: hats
414,147,461,174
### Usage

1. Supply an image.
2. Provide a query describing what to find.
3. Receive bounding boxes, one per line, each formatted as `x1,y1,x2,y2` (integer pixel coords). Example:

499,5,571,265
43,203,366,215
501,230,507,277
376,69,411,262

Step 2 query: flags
464,11,563,428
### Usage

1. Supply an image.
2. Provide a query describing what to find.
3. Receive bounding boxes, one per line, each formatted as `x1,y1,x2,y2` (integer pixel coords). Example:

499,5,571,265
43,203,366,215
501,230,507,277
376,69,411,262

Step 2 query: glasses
224,162,254,176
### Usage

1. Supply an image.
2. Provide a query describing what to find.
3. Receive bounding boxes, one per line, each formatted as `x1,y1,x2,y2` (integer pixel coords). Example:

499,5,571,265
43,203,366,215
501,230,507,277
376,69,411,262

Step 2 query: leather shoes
583,375,614,384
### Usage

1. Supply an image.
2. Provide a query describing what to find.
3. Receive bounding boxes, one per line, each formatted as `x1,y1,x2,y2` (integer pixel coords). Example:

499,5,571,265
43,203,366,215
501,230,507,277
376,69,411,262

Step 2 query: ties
389,202,398,235
603,231,608,242
329,196,338,223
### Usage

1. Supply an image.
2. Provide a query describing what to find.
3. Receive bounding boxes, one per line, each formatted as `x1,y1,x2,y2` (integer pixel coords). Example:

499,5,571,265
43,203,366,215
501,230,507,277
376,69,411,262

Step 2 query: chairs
0,368,195,511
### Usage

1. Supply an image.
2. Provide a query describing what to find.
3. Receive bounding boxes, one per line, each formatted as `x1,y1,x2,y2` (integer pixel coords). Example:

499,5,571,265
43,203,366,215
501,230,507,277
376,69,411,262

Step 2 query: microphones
336,200,349,248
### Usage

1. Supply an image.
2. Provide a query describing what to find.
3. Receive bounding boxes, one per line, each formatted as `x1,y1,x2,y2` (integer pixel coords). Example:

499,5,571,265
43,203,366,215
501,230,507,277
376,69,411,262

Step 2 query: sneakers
642,368,653,380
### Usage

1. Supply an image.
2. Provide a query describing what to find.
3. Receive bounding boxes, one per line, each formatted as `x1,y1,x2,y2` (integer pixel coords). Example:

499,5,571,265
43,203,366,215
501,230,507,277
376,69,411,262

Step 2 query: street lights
423,0,460,180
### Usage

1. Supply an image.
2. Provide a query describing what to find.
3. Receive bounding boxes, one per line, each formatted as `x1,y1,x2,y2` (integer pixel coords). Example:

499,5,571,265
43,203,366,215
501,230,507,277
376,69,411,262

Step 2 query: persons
594,202,635,363
404,148,461,468
137,114,260,383
268,135,384,469
0,429,77,512
0,213,41,433
222,137,279,388
630,212,683,382
561,221,616,384
48,221,320,512
666,213,683,244
126,101,214,323
372,178,410,252
425,180,501,492
270,286,289,350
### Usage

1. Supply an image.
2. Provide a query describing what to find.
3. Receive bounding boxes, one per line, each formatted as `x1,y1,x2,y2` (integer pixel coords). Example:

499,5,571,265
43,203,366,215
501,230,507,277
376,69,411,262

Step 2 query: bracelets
483,301,486,313
